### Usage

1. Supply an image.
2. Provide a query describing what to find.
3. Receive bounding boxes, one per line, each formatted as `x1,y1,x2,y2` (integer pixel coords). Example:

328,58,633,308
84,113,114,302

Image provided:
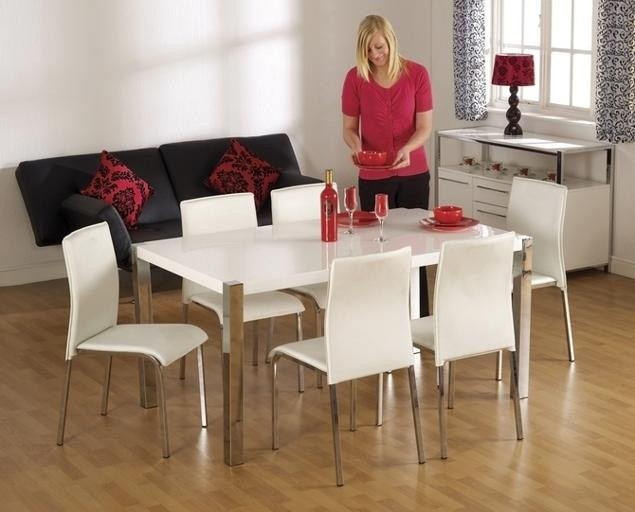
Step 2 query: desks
129,205,537,471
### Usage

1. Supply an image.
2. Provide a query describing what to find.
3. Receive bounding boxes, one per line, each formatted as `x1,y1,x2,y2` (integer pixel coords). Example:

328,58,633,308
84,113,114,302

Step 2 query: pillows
80,148,155,229
199,136,282,206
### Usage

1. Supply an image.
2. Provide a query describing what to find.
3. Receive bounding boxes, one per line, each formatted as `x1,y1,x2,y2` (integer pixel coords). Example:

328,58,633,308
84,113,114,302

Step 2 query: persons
341,14,434,318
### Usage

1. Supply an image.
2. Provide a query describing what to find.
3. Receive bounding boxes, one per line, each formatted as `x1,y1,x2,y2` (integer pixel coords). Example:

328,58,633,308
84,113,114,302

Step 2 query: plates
425,217,472,226
419,215,480,234
354,163,391,171
332,209,378,229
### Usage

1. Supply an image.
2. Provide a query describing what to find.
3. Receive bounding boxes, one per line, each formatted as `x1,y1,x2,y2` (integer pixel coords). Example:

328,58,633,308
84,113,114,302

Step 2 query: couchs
15,132,329,304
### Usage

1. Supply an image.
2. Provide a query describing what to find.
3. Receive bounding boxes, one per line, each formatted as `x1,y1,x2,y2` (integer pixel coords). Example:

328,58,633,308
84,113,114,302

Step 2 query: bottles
318,168,338,243
320,241,337,270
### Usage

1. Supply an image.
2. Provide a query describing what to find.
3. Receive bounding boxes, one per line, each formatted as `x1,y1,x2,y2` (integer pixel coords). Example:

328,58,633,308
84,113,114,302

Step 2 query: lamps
490,53,537,134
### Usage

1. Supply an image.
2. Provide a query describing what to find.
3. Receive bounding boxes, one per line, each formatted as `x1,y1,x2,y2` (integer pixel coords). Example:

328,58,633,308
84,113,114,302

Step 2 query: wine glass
373,194,391,242
342,186,359,236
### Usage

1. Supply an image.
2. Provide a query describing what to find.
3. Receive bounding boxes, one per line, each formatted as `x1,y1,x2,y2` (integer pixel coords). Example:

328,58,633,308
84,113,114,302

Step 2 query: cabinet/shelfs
432,123,619,280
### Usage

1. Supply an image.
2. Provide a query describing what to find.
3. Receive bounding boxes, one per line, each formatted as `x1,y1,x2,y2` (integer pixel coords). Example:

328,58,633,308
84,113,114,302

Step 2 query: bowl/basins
432,205,463,225
355,150,387,166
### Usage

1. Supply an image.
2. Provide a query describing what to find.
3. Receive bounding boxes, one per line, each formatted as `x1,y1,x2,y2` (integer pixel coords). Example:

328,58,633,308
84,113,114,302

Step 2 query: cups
457,154,568,185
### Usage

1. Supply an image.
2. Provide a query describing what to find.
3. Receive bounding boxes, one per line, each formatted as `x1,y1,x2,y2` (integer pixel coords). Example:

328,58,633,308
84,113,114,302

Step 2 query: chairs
491,174,577,386
57,221,209,460
177,192,306,395
266,244,426,485
373,229,527,461
270,182,340,391
